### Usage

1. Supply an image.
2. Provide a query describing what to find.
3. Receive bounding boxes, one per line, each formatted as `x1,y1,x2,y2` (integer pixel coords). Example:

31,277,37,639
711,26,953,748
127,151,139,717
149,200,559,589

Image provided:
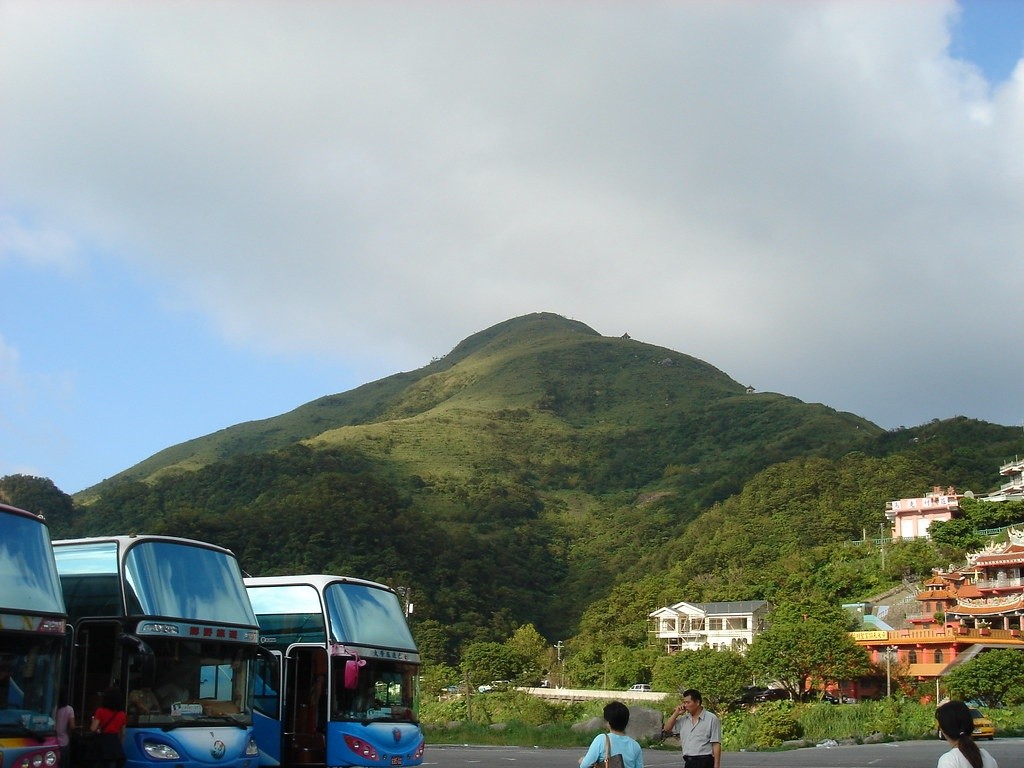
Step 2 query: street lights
886,645,898,697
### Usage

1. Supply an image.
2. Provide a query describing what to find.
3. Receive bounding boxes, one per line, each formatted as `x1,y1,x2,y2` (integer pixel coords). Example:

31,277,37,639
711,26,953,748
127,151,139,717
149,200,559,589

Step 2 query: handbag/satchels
95,728,103,737
592,733,623,768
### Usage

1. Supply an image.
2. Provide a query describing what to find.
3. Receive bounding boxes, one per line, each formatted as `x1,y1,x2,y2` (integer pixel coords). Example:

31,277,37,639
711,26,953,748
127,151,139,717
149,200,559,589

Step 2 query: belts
687,756,711,760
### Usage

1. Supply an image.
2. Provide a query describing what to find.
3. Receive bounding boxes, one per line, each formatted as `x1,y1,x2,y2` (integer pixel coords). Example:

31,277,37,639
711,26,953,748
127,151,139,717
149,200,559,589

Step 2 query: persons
577,701,644,768
935,701,999,768
664,689,721,768
55,685,75,768
310,660,379,734
89,673,191,768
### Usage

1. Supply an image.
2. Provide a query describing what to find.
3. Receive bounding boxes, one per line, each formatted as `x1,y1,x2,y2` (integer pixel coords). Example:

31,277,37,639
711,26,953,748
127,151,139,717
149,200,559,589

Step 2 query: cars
803,688,838,704
716,685,791,714
627,683,653,692
934,707,994,741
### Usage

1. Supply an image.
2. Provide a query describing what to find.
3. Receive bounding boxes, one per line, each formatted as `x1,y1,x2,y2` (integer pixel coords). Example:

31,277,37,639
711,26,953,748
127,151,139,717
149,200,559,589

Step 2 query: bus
203,573,426,768
0,500,84,768
49,532,279,768
